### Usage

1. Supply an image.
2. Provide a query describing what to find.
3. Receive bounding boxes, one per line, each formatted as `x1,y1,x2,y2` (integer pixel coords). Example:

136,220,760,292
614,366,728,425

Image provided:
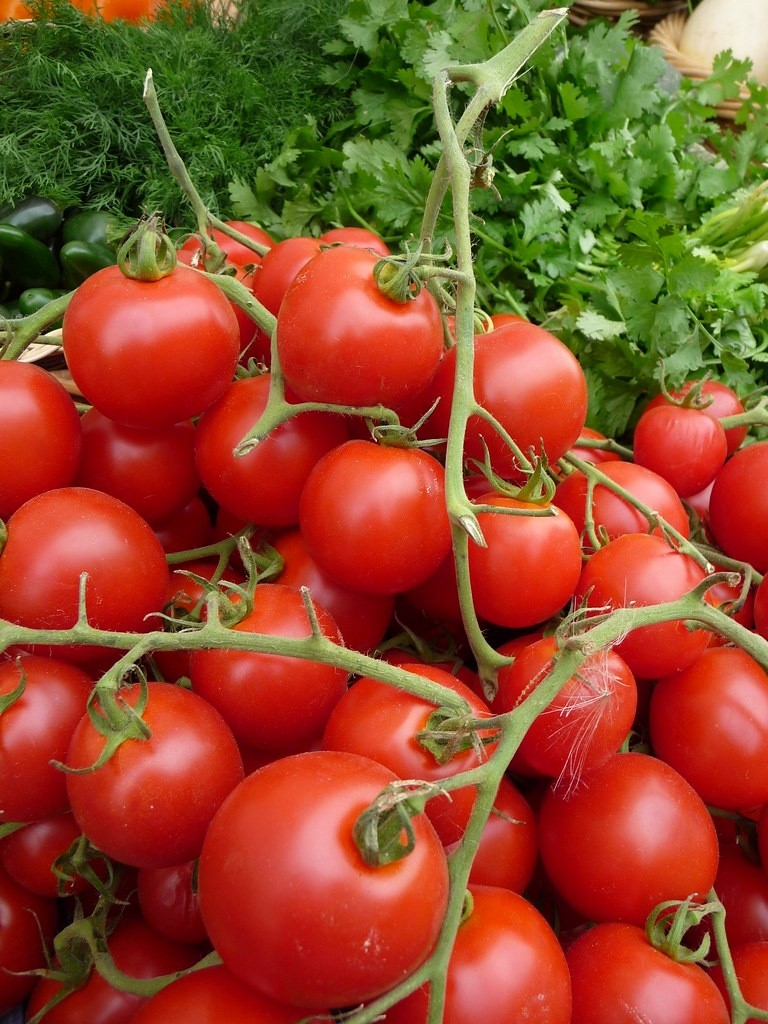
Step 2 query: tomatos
0,220,768,1024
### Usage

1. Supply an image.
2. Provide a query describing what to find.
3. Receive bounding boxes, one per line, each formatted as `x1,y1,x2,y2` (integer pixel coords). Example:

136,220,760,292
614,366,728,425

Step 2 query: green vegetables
0,0,768,446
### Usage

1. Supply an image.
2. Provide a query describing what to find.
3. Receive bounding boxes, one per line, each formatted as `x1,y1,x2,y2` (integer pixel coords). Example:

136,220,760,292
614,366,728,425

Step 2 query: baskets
561,0,687,45
650,11,768,161
